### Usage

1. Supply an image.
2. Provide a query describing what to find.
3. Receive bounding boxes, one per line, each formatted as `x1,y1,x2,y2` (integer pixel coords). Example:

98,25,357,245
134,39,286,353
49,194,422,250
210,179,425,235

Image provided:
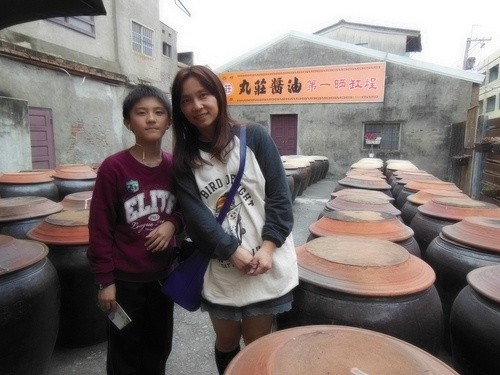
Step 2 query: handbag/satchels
165,241,211,312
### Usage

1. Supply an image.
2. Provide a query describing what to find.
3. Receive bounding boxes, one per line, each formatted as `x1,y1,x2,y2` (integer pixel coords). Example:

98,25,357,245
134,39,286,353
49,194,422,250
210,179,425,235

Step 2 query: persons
86,84,185,375
170,65,300,375
